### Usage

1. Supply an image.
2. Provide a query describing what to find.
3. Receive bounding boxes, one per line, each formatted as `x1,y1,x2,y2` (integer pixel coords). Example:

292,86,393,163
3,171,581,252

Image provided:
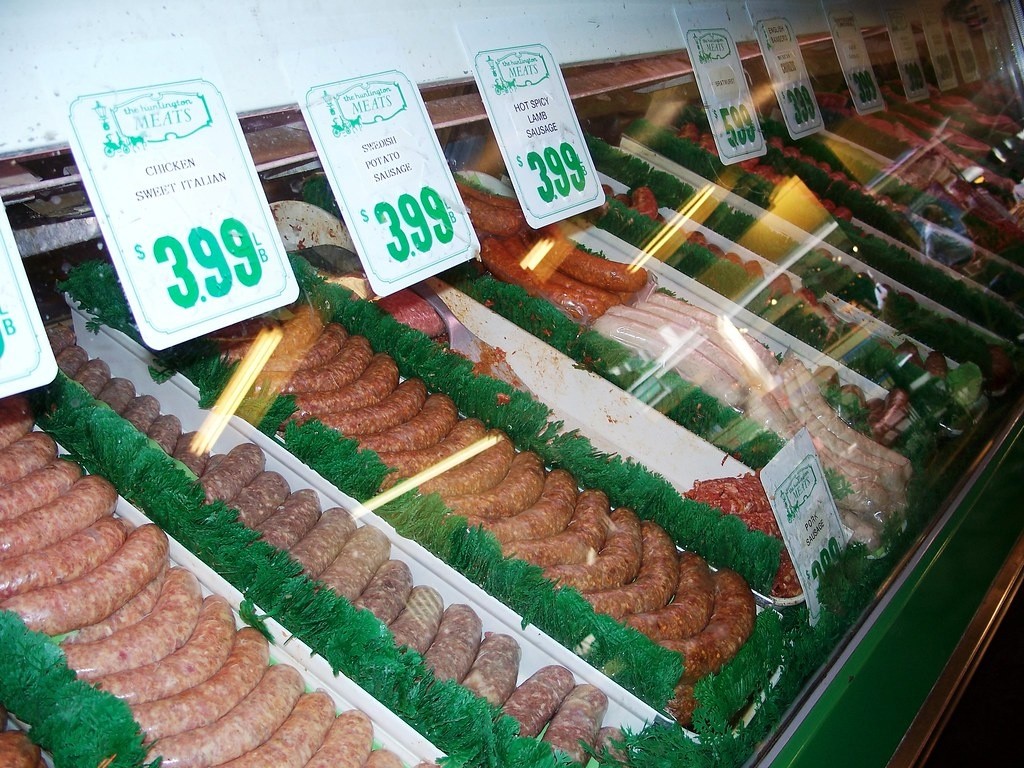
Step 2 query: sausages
0,78,1024,768
